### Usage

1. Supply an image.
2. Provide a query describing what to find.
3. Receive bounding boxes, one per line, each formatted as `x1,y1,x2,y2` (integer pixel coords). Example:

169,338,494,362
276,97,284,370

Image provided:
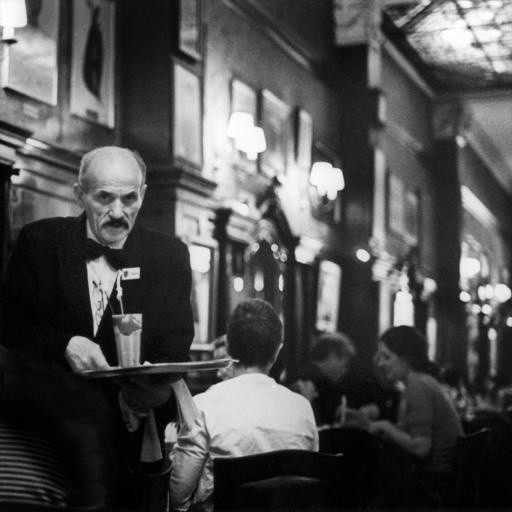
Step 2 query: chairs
213,449,346,512
456,427,493,476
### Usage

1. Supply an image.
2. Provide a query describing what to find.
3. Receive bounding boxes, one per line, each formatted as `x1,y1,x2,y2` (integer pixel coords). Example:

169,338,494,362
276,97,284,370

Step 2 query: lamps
235,126,267,161
225,112,254,140
0,0,28,45
309,161,333,196
321,167,345,205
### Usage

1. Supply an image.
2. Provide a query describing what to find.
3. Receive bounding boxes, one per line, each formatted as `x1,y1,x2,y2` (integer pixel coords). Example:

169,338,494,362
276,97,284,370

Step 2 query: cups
112,314,143,368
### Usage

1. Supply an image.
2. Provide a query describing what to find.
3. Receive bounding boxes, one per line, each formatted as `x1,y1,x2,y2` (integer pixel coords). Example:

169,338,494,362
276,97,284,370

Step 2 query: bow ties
82,237,141,271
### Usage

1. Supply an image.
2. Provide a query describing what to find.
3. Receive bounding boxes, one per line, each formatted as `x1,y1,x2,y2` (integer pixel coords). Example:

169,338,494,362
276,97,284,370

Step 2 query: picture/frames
171,58,204,172
183,232,219,351
177,0,204,65
314,259,342,335
294,103,316,174
69,0,116,131
0,0,60,108
257,87,295,182
227,71,259,130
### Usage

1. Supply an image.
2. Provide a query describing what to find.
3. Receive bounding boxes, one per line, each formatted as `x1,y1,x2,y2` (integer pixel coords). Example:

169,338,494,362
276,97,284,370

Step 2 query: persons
367,327,463,475
1,147,192,510
285,331,354,436
167,300,320,507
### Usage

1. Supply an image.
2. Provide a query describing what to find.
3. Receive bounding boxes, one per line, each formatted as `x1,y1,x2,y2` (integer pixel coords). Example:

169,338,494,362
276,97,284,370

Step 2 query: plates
78,360,237,380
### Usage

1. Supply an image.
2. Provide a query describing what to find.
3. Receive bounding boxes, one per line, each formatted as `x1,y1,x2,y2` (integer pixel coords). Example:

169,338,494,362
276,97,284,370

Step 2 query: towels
141,378,199,462
